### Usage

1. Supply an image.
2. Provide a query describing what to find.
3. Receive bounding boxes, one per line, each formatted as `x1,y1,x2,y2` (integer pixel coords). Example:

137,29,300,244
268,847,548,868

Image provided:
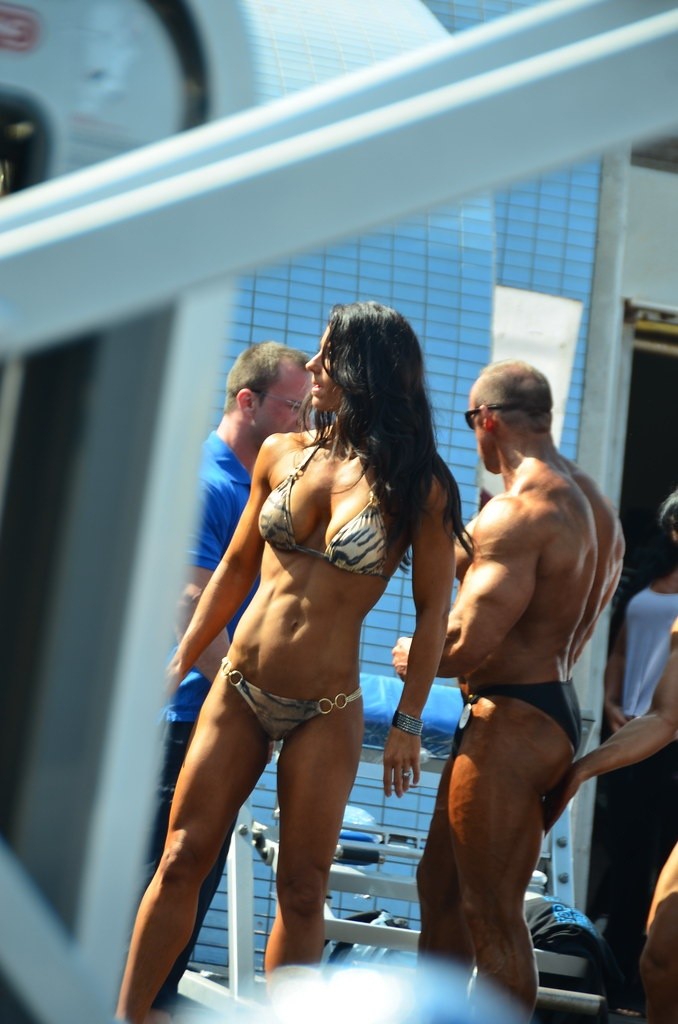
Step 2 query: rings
402,771,411,778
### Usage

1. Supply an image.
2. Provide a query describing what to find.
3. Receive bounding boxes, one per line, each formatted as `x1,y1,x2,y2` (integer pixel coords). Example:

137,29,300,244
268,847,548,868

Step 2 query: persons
601,487,677,1024
117,301,478,1024
141,339,312,1014
388,360,628,1023
537,617,677,1024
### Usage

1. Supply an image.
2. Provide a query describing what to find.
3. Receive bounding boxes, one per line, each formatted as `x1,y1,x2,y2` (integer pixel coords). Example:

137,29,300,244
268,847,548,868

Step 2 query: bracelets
391,711,424,735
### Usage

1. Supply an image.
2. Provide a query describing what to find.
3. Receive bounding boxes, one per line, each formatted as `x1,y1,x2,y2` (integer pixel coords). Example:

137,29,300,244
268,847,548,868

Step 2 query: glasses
465,404,501,430
254,390,313,415
